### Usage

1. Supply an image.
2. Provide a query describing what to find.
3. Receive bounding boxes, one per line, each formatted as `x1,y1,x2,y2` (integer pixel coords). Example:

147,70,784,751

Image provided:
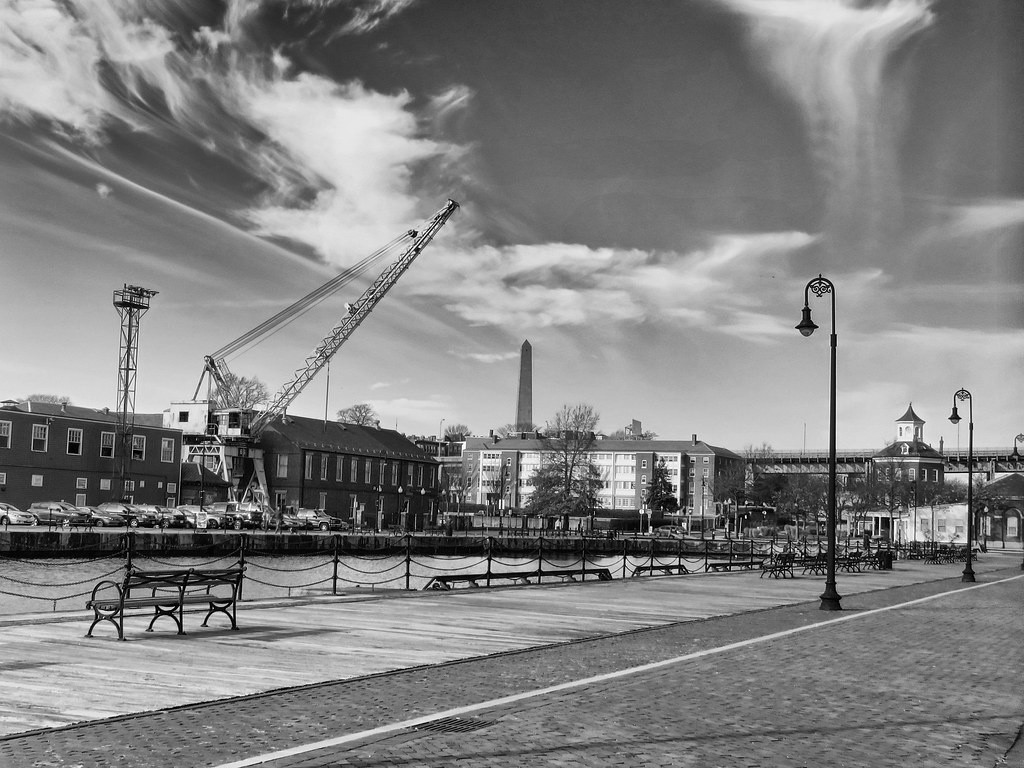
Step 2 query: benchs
802,553,828,575
835,551,863,573
924,546,977,565
83,567,249,643
760,552,797,579
863,551,886,571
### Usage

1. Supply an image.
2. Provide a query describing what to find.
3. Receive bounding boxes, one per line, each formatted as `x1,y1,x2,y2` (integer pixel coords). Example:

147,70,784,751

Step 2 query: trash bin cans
879,550,892,570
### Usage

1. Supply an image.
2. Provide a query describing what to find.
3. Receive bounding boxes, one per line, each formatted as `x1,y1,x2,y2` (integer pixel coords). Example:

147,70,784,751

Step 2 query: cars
76,506,125,527
297,509,342,531
177,506,227,529
98,502,160,528
268,513,312,531
134,505,187,528
654,525,688,538
0,503,35,525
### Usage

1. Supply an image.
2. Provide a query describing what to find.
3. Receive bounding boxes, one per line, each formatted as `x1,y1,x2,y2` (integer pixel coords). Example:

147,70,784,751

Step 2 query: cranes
182,198,459,510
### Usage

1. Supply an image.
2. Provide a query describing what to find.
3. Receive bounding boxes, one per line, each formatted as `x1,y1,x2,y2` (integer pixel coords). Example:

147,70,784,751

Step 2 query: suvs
213,501,263,529
27,502,92,526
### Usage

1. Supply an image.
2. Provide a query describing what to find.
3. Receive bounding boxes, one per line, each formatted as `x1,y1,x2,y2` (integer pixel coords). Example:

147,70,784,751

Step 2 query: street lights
948,387,975,582
1009,433,1024,459
794,273,842,610
